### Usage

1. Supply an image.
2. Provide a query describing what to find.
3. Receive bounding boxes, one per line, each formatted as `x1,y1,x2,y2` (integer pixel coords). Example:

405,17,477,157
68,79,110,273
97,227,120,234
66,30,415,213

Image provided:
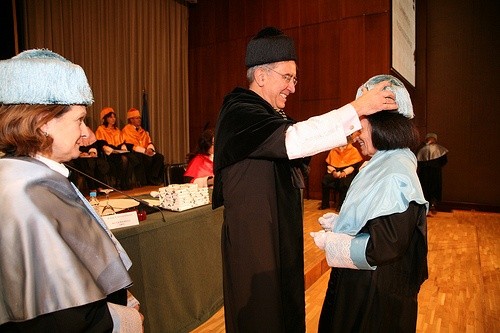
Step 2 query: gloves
310,230,331,251
318,212,339,232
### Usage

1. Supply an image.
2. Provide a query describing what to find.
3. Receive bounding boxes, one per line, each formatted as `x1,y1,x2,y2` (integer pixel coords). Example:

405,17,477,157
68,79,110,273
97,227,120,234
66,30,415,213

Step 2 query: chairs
164,163,189,185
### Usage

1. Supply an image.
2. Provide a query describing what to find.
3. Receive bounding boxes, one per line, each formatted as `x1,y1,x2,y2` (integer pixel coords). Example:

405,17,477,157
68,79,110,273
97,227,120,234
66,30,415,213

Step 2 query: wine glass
101,189,115,216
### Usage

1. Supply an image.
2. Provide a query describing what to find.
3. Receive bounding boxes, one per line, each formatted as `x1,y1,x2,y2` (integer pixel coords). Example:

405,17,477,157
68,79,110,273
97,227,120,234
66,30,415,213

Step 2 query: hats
245,28,297,68
126,108,140,120
355,74,415,120
0,48,94,105
427,133,437,140
100,107,114,122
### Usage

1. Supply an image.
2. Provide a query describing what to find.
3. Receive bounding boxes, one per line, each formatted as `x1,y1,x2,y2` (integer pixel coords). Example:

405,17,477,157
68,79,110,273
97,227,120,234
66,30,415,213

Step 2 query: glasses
260,66,297,87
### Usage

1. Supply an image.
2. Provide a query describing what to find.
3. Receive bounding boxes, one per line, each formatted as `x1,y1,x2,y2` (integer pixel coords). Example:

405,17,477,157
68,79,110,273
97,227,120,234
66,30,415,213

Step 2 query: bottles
88,191,100,215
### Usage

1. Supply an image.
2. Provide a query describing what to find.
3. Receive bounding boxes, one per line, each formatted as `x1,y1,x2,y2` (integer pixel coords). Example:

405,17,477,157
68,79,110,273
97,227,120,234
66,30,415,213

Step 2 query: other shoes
319,204,330,210
335,203,342,212
431,207,436,214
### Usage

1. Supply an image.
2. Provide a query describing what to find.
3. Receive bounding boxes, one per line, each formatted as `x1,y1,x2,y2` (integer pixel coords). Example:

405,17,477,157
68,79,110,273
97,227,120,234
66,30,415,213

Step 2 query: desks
101,188,227,333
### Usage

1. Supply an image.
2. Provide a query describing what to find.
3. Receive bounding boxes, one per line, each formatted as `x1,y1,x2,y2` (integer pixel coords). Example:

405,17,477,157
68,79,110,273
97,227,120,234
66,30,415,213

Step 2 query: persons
417,133,448,215
122,107,164,186
95,107,133,191
0,48,144,333
211,25,400,333
310,74,429,333
318,140,363,212
62,125,109,197
179,131,215,190
